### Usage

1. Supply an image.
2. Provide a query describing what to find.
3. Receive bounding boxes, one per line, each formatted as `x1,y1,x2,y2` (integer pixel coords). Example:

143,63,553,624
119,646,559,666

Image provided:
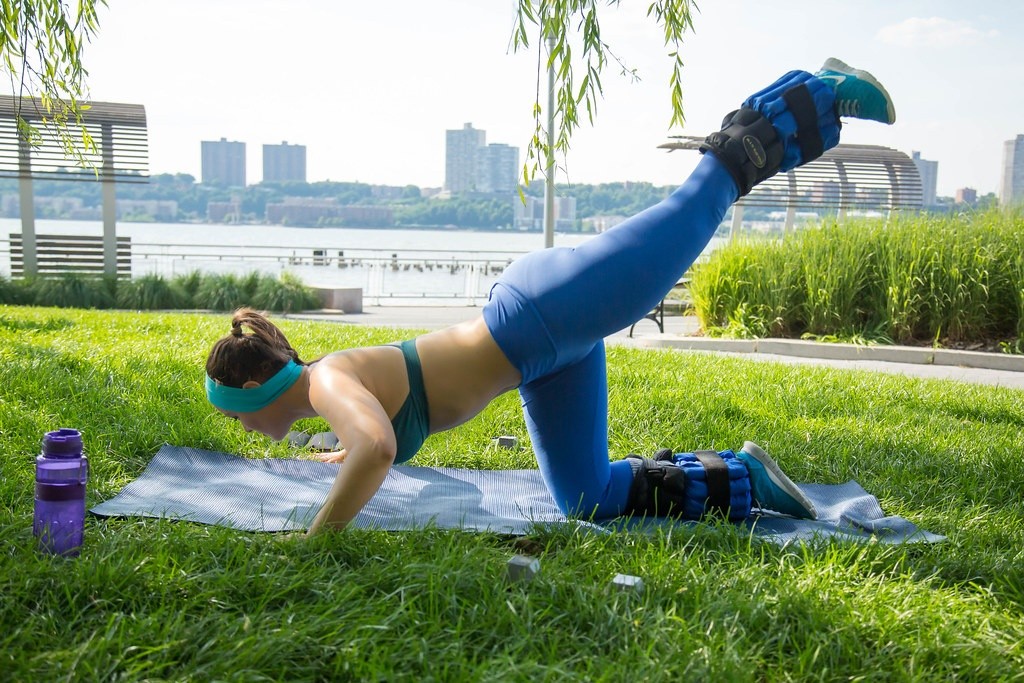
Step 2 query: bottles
34,429,87,557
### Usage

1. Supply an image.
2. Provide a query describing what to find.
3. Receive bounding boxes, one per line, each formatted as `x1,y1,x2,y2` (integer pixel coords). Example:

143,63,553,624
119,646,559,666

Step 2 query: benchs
629,298,664,337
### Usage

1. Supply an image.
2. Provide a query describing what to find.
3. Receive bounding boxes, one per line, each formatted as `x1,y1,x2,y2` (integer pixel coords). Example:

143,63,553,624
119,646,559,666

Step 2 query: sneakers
813,58,895,124
737,441,818,520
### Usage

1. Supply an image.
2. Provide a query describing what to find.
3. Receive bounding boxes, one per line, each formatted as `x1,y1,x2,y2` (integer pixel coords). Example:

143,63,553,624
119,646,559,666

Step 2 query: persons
204,58,896,547
